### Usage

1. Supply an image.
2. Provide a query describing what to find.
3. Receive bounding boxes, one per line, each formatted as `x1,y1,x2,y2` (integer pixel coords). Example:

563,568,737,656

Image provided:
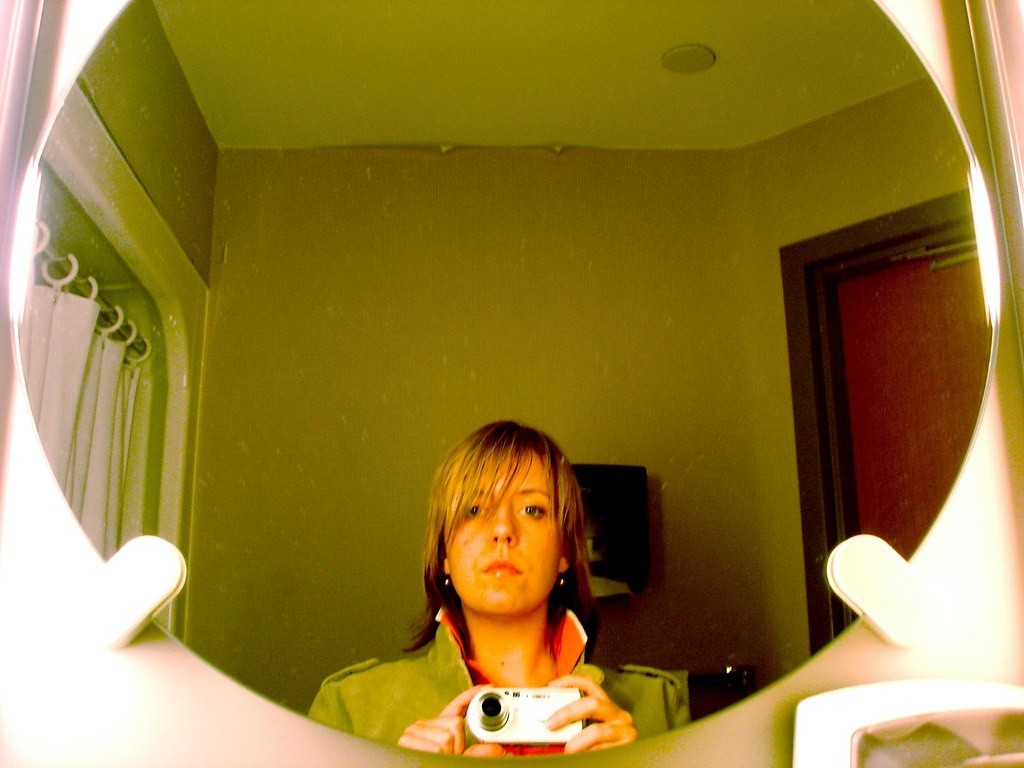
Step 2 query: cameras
466,687,582,746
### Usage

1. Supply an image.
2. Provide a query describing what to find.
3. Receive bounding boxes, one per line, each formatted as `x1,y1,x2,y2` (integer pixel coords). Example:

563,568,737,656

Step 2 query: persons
306,420,690,759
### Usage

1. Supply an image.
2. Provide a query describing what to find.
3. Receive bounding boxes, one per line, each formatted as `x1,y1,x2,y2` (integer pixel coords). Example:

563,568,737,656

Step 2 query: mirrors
11,0,1004,767
